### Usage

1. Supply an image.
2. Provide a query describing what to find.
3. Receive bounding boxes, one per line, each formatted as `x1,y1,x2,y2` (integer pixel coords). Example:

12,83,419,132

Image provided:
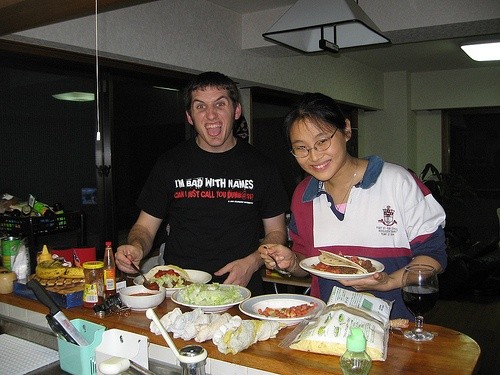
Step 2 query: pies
145,265,190,281
317,249,368,274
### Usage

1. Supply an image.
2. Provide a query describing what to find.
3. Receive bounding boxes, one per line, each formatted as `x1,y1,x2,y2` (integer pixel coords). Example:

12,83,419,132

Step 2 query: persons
258,93,447,321
114,70,289,299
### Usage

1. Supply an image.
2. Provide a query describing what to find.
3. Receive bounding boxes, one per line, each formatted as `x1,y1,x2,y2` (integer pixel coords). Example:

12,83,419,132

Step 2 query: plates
299,253,386,280
239,293,326,326
133,267,212,298
169,283,252,313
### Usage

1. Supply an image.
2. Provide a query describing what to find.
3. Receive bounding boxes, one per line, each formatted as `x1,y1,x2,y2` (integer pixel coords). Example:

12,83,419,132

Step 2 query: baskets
0,208,83,251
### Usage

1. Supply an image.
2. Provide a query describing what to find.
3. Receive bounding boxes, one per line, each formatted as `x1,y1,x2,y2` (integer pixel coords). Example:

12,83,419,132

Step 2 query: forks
264,246,295,280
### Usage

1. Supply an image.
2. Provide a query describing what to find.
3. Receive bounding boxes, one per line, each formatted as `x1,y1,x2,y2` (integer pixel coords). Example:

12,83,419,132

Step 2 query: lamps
459,38,500,62
262,0,392,56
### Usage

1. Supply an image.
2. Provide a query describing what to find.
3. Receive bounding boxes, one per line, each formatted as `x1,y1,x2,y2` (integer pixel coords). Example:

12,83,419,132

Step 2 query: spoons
123,254,160,291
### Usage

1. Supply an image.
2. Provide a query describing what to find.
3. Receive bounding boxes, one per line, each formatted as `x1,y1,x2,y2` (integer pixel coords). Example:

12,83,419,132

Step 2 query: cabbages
148,274,187,288
182,282,244,306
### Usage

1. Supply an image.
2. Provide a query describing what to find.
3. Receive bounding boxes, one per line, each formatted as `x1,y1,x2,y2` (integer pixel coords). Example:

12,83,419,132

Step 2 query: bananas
35,260,85,279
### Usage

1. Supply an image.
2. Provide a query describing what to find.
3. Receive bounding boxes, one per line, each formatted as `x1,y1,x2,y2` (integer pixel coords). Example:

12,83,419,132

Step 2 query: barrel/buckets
0,235,31,281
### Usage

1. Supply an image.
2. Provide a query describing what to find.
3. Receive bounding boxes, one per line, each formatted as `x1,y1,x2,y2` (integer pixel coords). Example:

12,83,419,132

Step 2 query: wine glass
401,263,440,340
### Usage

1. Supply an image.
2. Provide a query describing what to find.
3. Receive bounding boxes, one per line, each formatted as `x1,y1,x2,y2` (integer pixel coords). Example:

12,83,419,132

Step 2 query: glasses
289,125,339,157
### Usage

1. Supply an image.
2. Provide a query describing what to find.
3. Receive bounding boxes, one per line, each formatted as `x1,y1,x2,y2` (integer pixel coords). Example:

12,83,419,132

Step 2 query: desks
260,272,313,295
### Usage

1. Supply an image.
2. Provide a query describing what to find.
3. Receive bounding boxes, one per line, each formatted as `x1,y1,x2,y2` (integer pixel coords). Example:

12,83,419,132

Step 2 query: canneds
83,261,105,308
0,237,21,270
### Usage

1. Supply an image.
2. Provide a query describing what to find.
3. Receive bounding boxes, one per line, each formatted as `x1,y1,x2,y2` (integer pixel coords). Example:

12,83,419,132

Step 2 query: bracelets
288,254,298,273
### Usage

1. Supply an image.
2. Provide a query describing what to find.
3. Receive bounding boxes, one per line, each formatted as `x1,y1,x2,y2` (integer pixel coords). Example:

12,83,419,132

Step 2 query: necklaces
337,160,360,211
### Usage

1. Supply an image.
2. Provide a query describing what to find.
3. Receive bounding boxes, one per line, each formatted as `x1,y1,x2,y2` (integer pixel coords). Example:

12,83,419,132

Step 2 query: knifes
26,279,88,347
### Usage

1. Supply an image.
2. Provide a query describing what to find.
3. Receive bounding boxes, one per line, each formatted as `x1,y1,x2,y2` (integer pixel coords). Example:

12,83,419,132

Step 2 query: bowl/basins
119,284,166,313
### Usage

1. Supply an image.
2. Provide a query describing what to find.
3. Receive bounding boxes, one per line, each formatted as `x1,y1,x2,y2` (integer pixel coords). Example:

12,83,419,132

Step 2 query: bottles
178,344,208,375
339,326,373,375
102,241,116,298
